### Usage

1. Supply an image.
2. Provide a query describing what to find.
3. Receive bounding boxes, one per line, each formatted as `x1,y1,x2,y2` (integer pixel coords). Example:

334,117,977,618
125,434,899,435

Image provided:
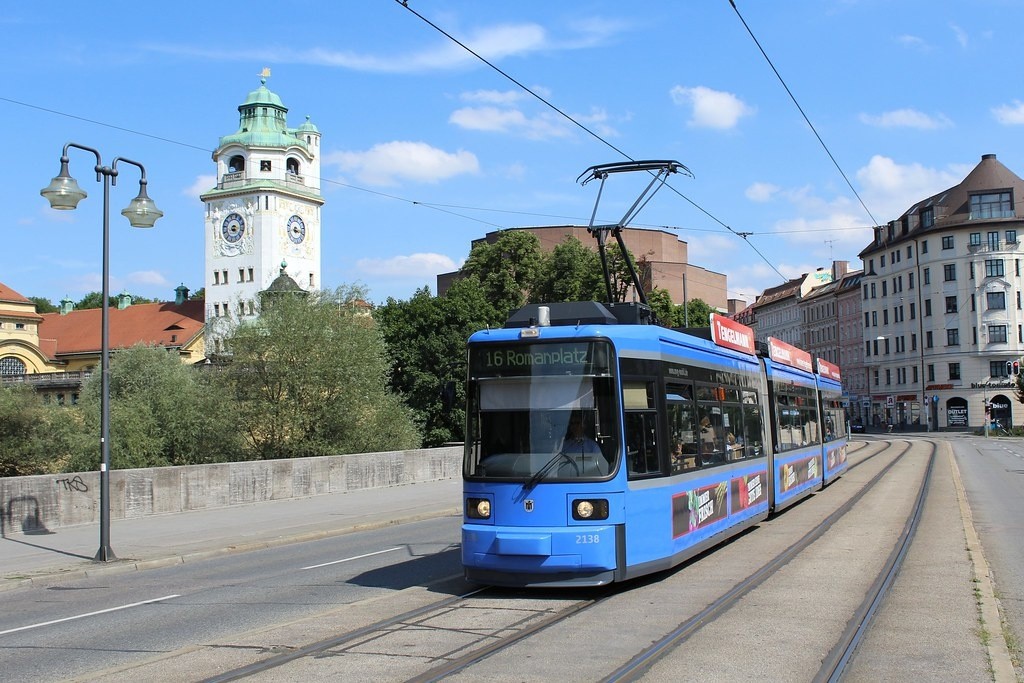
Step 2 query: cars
851,422,865,433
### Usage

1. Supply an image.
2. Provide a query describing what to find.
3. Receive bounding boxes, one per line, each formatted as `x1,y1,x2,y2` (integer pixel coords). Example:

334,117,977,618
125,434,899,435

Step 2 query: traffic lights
1013,361,1019,375
1006,362,1012,375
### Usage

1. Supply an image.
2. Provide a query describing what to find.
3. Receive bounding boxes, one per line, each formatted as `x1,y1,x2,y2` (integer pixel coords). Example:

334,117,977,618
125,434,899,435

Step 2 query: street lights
40,141,164,565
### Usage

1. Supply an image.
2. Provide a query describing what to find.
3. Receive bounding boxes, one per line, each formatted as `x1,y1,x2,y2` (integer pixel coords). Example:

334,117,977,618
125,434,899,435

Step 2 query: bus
461,301,846,588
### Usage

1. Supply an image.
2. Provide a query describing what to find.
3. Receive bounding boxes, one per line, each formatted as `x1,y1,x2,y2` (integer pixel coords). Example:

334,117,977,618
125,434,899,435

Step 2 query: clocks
286,216,305,245
221,213,246,245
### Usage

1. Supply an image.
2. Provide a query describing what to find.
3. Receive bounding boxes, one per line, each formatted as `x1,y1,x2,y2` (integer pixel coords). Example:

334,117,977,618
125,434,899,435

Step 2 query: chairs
729,444,742,459
677,457,695,471
